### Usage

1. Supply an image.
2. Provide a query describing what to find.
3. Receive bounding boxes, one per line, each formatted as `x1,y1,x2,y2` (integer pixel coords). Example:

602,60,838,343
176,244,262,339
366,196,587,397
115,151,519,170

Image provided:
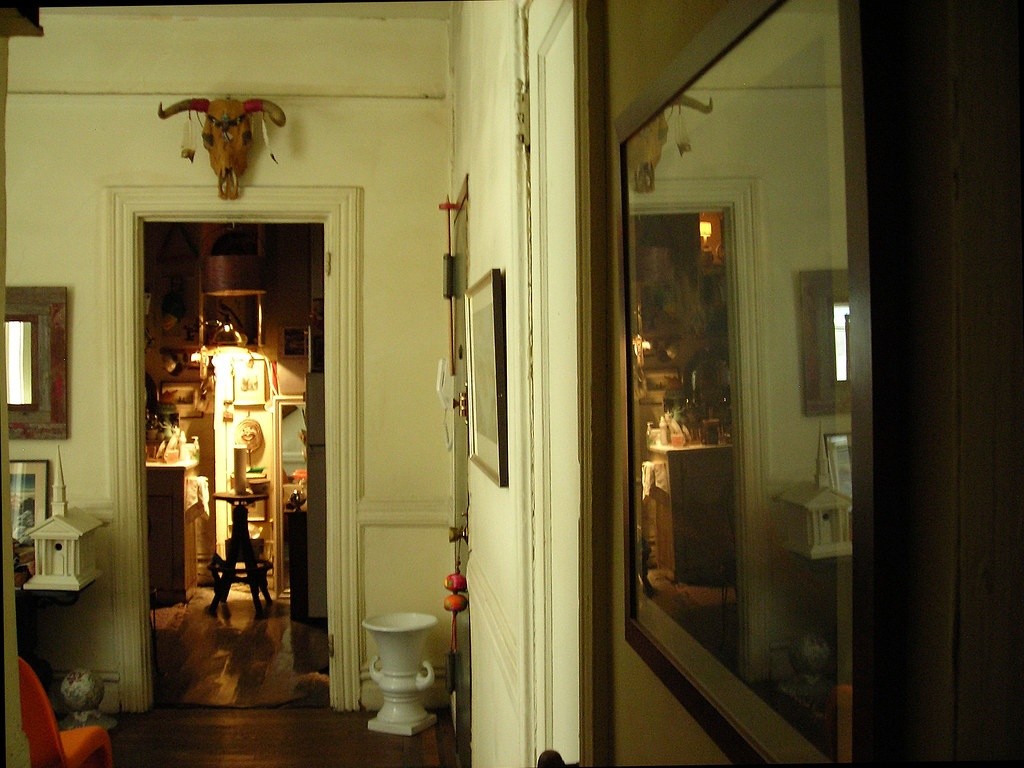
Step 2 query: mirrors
6,286,70,440
613,1,876,768
798,269,855,417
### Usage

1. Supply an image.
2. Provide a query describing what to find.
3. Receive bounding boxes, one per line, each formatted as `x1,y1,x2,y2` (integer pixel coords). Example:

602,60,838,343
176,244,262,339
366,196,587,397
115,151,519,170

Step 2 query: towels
184,475,210,525
641,460,669,506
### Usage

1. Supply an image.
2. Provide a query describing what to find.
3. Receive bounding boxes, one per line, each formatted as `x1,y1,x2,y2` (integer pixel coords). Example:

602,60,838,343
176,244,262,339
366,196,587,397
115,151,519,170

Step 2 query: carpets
149,585,329,708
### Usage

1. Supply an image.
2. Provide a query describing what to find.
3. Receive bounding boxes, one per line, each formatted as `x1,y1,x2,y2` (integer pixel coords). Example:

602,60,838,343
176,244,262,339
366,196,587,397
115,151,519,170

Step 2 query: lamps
208,306,242,344
202,256,269,297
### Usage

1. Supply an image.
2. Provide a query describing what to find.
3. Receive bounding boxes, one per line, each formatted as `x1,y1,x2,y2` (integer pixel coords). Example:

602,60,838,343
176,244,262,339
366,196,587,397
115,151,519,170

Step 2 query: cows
624,93,714,194
158,97,287,200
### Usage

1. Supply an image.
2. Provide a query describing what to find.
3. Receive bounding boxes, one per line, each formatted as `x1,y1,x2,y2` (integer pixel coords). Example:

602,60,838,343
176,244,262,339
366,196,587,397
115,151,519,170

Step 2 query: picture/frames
8,460,48,581
469,267,507,487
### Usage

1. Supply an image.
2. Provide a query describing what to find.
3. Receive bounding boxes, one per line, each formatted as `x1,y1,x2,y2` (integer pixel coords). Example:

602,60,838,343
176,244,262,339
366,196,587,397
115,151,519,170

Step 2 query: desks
209,488,273,618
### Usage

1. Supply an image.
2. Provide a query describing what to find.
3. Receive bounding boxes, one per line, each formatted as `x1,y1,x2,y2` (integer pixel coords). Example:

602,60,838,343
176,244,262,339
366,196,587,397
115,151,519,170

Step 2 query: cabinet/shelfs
197,291,328,619
146,458,197,605
647,441,739,591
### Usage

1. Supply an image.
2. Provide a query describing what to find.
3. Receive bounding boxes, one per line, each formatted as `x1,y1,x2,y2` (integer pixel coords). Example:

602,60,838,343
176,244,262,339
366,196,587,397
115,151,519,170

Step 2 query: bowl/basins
249,479,270,494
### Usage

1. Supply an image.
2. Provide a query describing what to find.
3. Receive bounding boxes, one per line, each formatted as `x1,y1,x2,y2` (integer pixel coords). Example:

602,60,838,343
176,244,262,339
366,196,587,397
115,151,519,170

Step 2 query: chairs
19,657,112,768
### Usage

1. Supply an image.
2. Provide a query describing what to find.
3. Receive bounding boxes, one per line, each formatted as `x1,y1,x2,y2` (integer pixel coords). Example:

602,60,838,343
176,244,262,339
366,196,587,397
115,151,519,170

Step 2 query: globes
61,668,117,733
